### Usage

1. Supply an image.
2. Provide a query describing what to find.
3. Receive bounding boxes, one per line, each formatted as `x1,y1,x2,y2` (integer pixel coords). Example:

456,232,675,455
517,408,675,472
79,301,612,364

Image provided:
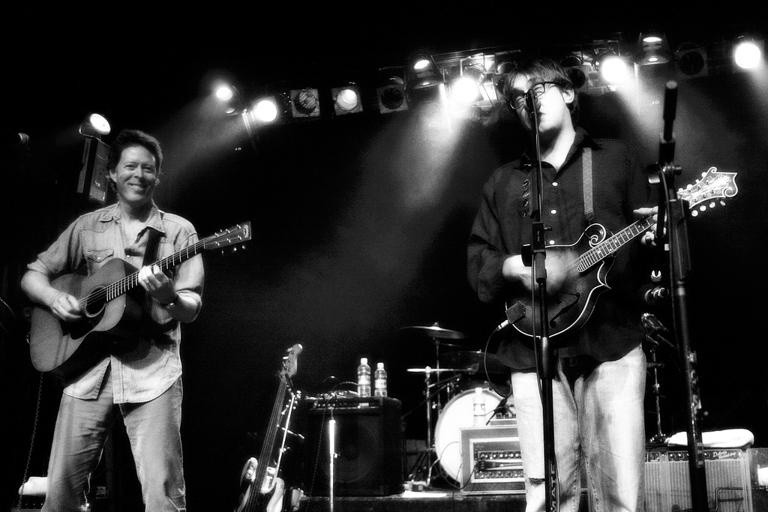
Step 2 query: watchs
158,293,181,313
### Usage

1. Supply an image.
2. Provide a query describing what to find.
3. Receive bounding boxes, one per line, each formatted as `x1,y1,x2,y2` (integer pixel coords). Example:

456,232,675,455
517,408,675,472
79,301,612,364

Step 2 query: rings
144,281,151,285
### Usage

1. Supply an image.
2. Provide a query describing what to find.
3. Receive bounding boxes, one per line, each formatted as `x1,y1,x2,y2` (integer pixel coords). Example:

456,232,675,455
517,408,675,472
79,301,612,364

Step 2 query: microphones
640,312,667,338
661,80,680,141
524,91,542,116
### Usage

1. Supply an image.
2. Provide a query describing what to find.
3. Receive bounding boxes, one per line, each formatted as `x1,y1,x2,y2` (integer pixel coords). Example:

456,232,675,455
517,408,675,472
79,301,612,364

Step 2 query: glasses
509,81,565,110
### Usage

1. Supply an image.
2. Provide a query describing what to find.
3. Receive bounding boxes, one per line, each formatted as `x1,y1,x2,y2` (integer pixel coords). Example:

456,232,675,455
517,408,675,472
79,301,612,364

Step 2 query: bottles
373,361,388,399
357,356,372,399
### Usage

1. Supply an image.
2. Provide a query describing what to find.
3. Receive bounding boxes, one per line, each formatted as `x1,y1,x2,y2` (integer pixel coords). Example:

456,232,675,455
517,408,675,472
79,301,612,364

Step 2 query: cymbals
406,326,465,339
460,351,501,363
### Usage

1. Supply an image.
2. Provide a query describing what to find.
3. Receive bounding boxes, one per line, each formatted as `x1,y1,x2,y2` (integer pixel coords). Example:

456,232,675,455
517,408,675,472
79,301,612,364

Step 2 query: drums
434,386,516,482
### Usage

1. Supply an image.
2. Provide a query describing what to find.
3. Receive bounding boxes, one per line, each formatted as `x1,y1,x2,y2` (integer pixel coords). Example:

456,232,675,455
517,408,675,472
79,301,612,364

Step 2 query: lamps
205,28,767,135
78,114,114,143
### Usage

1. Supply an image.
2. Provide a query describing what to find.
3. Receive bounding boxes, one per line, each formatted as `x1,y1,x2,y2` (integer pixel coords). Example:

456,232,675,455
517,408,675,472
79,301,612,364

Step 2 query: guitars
503,167,739,340
30,221,251,381
233,344,304,512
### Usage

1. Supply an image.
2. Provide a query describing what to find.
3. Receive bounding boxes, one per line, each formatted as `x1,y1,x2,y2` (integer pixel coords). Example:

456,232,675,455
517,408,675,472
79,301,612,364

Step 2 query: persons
463,56,673,512
19,130,207,512
